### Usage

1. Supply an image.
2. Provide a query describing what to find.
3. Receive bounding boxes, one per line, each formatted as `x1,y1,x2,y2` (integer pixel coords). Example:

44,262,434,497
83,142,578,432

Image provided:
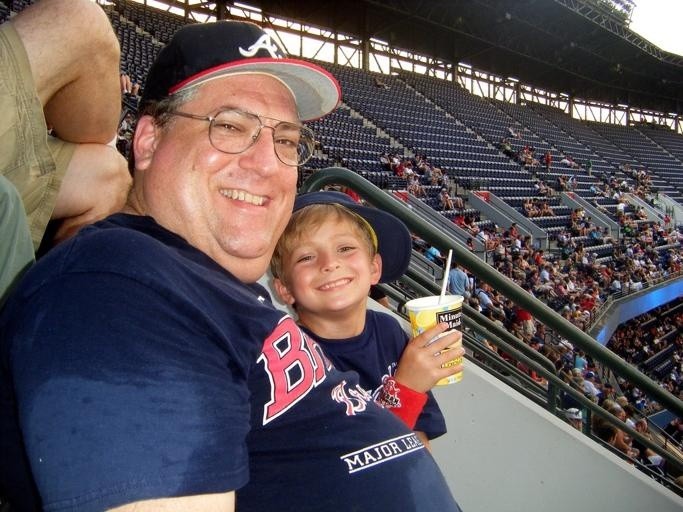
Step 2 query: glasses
164,106,316,166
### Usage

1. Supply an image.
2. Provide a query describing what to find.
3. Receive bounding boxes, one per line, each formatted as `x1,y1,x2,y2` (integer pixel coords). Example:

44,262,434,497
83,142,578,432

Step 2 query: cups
405,295,464,384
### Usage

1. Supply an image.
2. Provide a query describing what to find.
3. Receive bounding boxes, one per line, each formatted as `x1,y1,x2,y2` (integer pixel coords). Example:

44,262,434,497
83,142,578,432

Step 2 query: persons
0,21,463,512
1,0,132,257
269,118,683,497
115,66,142,172
373,75,390,89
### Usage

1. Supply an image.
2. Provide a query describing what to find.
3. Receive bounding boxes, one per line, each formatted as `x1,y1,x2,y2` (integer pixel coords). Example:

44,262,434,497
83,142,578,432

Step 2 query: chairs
102,0,615,349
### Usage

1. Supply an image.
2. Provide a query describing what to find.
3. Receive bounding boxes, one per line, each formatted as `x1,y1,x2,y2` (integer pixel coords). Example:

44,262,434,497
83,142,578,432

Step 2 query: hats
142,20,341,121
567,407,582,420
292,190,411,284
586,371,596,378
